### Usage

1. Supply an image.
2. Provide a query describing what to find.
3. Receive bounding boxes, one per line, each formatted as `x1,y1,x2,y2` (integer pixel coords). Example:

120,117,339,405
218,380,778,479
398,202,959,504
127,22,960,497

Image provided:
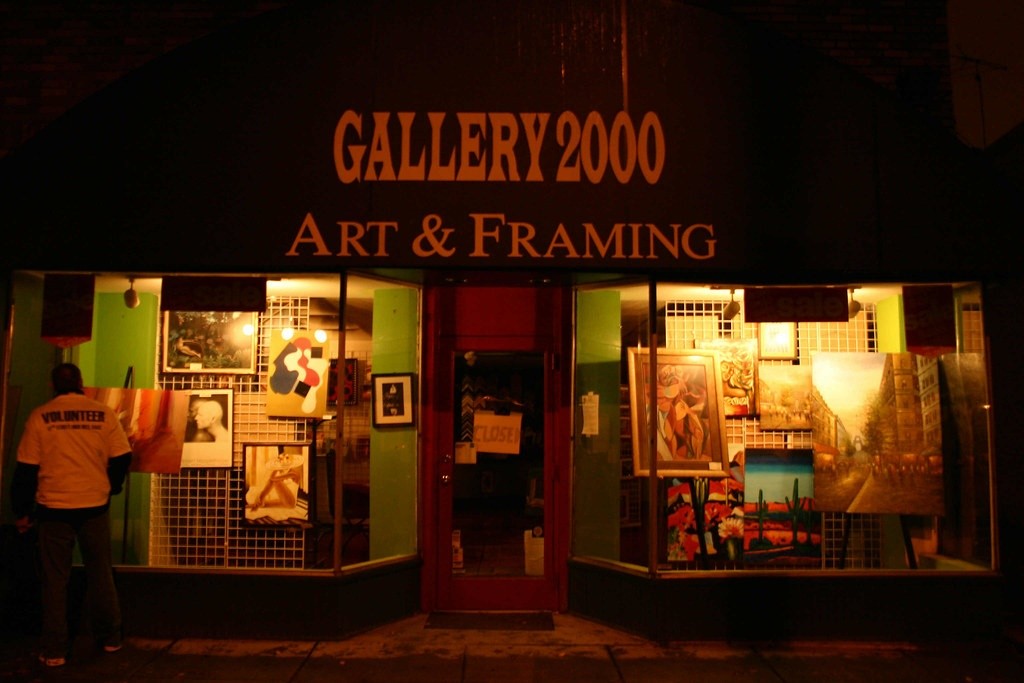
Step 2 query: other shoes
103,641,122,652
38,651,65,667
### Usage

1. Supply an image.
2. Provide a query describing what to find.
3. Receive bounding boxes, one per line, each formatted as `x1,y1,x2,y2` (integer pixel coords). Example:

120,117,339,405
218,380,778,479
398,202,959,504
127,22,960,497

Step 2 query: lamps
124,278,140,309
722,287,741,320
847,287,861,319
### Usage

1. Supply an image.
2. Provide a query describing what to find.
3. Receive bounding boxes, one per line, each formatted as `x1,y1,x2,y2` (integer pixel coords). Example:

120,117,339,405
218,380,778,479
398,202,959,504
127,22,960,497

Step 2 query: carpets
423,610,556,633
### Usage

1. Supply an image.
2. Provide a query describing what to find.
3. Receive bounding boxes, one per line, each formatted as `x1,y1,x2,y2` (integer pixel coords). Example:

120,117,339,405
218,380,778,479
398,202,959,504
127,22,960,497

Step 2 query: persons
186,399,228,443
8,363,133,669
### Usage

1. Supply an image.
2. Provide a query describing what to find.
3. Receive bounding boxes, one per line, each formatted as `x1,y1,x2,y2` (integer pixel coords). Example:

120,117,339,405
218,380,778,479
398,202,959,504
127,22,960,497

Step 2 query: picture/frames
241,440,313,531
627,347,730,478
162,309,259,375
370,371,416,429
328,359,359,407
